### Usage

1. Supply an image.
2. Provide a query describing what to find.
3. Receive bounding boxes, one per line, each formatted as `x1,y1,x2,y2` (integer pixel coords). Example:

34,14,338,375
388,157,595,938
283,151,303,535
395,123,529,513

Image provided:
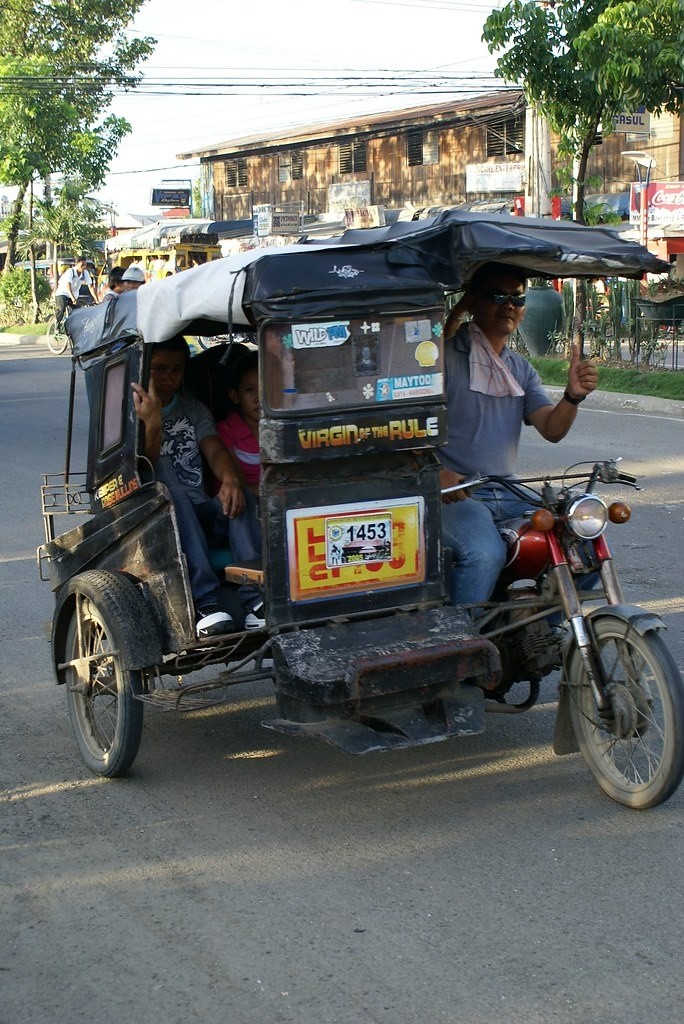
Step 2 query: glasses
491,293,526,306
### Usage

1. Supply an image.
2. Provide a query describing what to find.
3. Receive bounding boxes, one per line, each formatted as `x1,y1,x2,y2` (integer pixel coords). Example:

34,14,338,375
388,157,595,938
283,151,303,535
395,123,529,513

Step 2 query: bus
97,232,224,304
14,257,98,308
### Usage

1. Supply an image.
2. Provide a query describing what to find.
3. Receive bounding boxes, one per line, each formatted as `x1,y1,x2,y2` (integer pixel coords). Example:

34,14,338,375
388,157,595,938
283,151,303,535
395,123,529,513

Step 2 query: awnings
104,216,253,250
561,191,630,221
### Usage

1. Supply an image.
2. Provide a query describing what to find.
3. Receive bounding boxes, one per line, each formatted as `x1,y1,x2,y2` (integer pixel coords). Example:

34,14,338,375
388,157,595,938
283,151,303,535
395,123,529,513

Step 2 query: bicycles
46,299,99,355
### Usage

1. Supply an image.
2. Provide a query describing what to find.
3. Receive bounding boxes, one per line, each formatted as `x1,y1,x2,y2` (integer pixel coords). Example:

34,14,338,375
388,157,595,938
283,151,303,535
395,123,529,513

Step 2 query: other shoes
196,605,234,637
245,601,267,629
54,328,60,334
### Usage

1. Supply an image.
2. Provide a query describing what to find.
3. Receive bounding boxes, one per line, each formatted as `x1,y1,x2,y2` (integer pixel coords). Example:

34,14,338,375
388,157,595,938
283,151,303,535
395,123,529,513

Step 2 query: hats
121,267,145,282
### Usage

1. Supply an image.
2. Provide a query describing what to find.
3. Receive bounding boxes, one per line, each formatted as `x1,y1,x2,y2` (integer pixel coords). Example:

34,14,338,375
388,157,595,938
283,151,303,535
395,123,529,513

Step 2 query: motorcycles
37,209,684,810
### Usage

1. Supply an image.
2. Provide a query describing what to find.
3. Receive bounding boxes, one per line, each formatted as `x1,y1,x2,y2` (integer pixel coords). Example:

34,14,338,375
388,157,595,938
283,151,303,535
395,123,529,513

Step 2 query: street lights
622,151,657,295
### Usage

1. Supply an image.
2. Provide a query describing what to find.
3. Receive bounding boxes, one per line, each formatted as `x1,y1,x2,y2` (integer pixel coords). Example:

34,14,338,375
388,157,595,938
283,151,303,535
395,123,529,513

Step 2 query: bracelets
451,313,464,321
564,389,586,404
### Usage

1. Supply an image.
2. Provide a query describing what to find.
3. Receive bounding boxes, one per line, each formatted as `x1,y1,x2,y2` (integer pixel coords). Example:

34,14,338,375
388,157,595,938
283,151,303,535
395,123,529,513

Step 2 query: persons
53,256,146,335
440,261,614,666
130,336,284,637
146,256,198,268
